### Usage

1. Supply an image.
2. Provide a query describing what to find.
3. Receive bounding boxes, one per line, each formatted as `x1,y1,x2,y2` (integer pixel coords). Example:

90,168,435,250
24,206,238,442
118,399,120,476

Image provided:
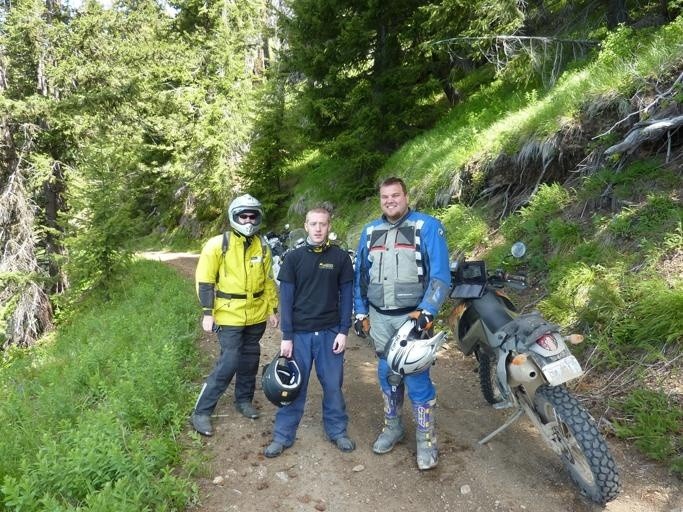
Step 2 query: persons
190,193,280,436
263,207,355,458
352,178,453,471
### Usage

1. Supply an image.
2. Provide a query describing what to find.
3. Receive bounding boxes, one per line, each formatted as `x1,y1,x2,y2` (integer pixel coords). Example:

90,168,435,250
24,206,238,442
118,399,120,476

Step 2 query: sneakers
265,442,288,458
336,435,356,451
190,411,214,437
235,403,258,418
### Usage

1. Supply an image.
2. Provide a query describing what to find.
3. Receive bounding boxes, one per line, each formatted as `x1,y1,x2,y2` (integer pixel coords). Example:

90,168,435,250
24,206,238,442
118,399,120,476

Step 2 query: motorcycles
260,222,367,341
446,239,623,503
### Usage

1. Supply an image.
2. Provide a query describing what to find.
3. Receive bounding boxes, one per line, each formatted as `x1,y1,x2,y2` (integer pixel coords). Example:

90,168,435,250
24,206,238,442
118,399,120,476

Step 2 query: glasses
240,214,257,219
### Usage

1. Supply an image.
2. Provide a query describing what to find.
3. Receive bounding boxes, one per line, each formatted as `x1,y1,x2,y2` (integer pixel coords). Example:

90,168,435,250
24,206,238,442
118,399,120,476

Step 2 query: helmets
262,351,302,406
383,317,447,376
228,194,264,237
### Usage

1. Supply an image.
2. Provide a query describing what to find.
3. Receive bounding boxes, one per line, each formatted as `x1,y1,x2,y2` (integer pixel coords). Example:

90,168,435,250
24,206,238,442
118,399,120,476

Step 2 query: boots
412,400,439,470
371,390,405,454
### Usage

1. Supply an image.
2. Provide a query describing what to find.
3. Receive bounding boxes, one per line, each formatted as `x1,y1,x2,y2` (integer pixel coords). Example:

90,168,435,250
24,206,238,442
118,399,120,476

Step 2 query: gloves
355,314,368,338
407,309,433,333
